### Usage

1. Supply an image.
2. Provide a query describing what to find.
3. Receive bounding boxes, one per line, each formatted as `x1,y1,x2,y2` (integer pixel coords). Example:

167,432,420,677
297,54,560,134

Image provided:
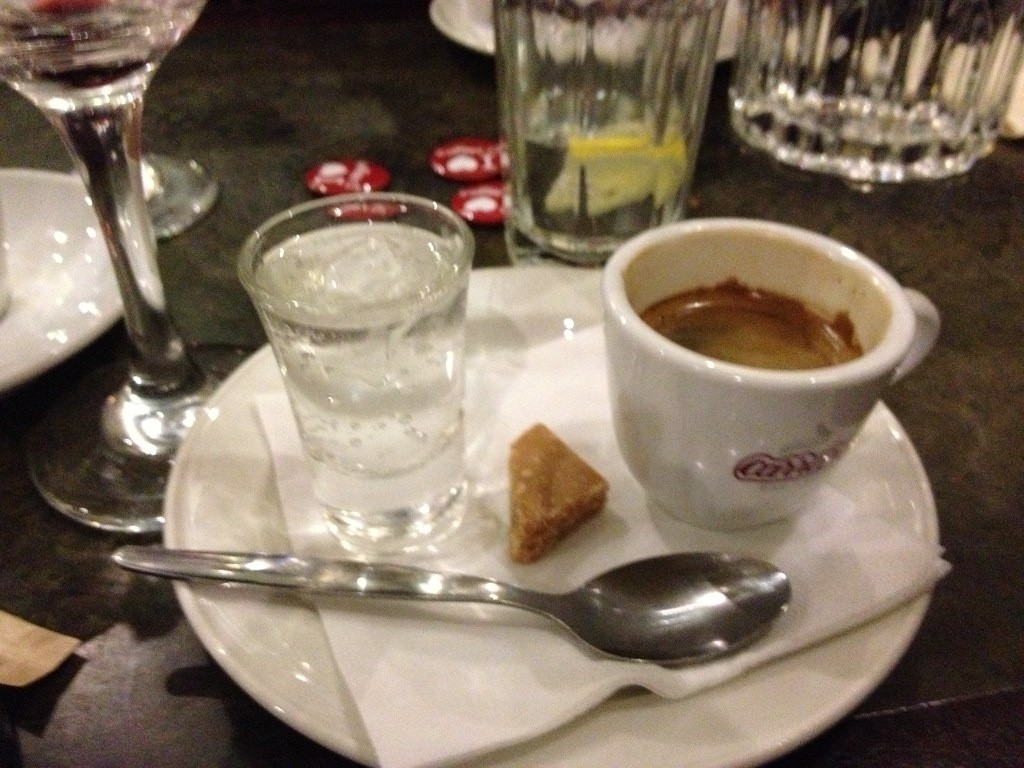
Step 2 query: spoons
110,544,792,666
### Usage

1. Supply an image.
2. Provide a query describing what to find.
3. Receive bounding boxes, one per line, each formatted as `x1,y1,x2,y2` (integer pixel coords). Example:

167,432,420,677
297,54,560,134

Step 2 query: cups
493,0,729,271
729,0,1024,184
599,217,942,531
236,192,475,556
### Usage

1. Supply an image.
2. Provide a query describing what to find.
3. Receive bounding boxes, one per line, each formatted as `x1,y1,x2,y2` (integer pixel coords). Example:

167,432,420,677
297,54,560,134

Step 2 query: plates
0,167,125,394
427,0,744,62
164,263,940,768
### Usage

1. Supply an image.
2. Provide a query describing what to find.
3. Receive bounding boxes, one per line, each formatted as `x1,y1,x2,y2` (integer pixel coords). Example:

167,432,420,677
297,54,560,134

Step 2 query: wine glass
69,151,218,242
0,0,259,534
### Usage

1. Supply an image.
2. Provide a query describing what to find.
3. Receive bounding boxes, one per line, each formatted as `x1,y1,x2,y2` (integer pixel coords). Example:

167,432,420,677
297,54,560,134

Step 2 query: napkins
256,319,955,768
